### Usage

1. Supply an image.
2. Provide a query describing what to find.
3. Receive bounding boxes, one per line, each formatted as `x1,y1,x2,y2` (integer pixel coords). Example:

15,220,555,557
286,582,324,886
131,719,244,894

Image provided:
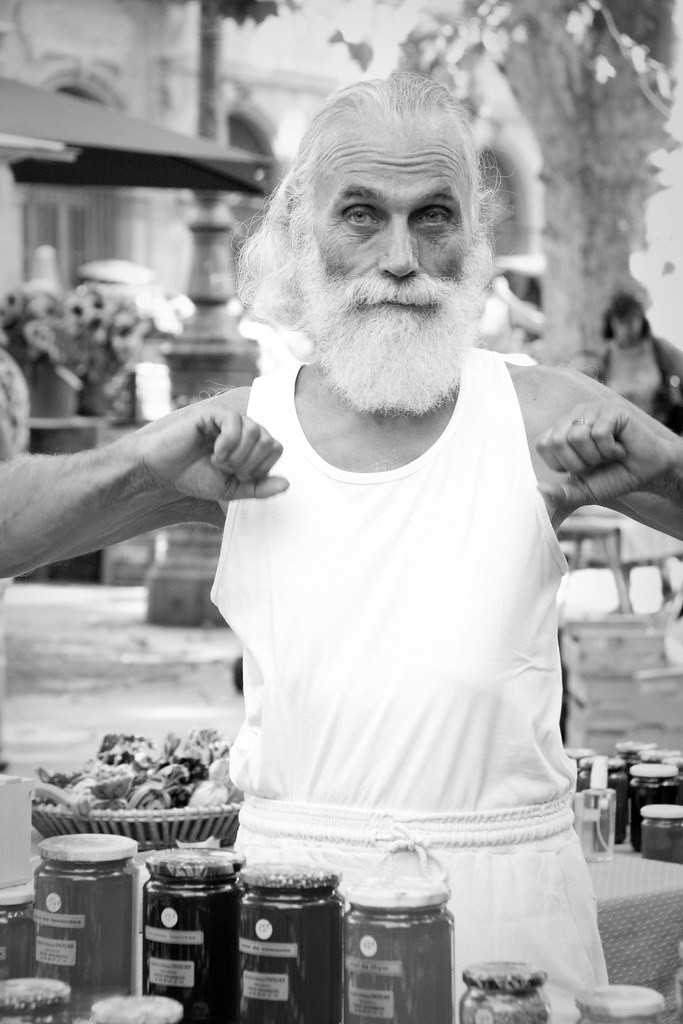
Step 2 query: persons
0,77,683,1024
589,295,683,612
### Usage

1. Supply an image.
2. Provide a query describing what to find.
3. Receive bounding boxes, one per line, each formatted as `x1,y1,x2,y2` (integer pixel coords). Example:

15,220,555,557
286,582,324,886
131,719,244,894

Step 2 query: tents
0,75,268,195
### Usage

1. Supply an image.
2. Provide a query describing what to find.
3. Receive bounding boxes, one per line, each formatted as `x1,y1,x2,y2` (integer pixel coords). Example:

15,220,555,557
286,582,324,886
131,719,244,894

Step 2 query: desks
583,836,683,1023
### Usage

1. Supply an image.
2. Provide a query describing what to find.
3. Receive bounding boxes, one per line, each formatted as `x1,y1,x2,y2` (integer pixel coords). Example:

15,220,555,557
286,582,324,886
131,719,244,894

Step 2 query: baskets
31,797,243,853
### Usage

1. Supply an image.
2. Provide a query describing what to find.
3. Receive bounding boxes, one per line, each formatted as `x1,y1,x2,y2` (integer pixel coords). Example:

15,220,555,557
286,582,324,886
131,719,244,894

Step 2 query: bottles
584,755,617,863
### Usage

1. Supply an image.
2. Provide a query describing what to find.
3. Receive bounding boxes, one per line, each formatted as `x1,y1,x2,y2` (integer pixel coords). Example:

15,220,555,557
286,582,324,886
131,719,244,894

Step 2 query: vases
26,361,82,416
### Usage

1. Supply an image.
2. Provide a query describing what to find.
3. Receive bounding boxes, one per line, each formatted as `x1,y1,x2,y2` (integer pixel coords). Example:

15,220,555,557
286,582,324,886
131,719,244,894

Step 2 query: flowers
0,281,152,381
